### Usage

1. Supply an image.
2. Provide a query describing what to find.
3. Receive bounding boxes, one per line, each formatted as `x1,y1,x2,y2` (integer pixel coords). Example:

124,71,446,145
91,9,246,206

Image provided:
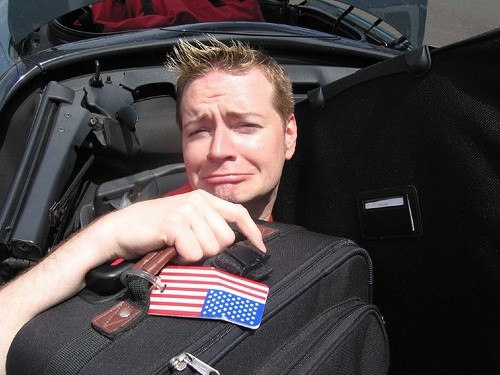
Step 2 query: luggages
6,217,394,375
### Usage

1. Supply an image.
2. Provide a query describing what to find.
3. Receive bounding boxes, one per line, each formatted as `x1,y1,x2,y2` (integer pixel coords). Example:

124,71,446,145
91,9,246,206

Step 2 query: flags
149,264,271,330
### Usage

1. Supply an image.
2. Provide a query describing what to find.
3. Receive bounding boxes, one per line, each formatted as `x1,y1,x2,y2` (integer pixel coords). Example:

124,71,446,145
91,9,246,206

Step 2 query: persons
0,34,298,375
